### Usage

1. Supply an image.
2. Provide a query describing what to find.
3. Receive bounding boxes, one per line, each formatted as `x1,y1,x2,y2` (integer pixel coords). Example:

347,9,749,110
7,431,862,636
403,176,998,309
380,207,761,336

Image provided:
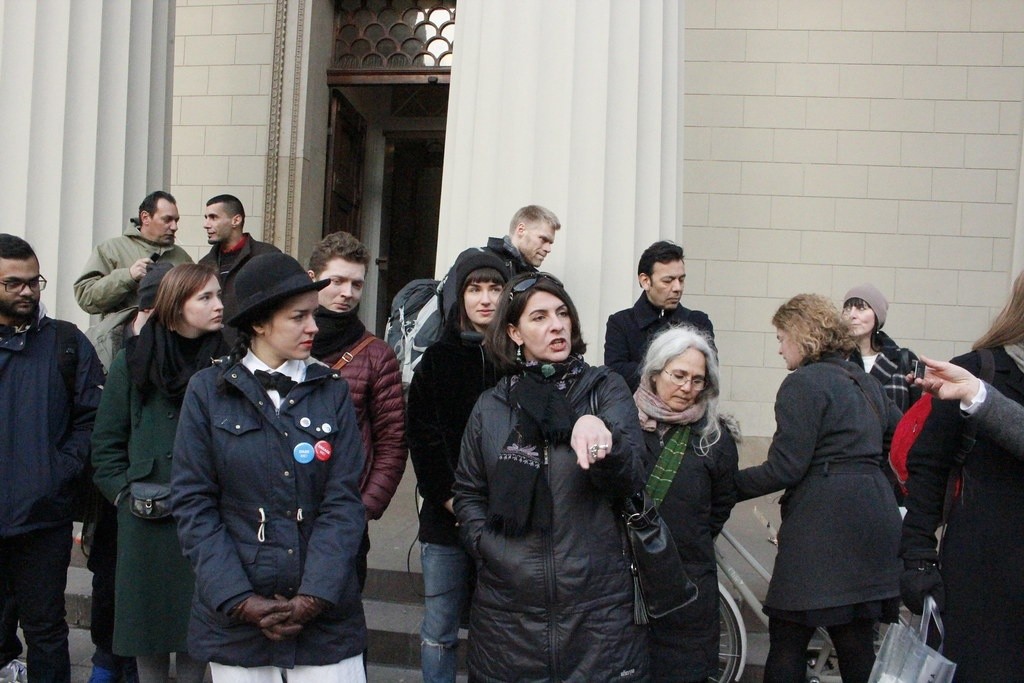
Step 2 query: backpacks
883,347,995,509
382,275,449,405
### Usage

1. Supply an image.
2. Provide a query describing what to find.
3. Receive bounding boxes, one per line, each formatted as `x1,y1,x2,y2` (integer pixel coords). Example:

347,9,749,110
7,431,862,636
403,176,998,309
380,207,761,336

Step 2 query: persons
309,231,408,592
900,269,1024,683
73,192,194,322
840,284,921,501
89,263,230,683
405,250,512,683
81,264,174,683
198,194,283,350
443,205,561,325
451,272,650,683
634,323,739,683
0,234,106,683
735,293,902,683
169,251,370,683
604,240,714,396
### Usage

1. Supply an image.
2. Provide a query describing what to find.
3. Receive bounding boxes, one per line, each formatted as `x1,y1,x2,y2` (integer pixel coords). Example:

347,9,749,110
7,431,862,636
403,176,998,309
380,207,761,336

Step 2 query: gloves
899,549,947,616
230,594,330,642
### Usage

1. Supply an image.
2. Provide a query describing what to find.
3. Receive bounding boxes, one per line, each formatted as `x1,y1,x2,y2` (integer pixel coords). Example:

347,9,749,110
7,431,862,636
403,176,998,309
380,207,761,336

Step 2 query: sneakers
0,659,28,683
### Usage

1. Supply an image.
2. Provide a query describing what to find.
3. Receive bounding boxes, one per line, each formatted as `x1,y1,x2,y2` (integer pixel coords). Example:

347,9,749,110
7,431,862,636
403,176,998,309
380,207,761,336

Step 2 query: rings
590,444,598,459
599,444,608,450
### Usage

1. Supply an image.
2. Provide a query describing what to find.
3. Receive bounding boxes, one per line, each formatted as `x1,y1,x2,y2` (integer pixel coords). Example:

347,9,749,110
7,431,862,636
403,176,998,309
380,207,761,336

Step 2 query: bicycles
708,500,923,683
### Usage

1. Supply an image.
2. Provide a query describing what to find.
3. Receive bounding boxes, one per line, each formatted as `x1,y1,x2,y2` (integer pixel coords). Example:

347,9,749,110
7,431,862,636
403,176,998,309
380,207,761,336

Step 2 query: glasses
0,274,47,294
509,272,564,302
662,367,708,391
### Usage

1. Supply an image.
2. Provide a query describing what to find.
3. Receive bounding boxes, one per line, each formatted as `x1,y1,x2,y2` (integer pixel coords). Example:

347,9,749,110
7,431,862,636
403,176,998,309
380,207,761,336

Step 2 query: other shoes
90,661,117,683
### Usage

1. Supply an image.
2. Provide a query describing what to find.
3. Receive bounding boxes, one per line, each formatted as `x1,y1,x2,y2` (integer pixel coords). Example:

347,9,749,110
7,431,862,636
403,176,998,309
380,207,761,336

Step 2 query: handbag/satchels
622,486,699,626
868,596,957,683
129,481,174,519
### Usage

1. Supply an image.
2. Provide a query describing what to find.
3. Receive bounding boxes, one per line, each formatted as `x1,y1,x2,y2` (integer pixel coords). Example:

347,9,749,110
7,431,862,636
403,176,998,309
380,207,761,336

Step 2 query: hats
455,252,510,299
221,251,331,325
842,281,889,332
136,261,175,312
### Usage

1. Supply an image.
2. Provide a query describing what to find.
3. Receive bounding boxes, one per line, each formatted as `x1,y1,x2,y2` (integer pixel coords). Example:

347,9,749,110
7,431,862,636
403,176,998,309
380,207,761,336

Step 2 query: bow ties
251,370,297,399
